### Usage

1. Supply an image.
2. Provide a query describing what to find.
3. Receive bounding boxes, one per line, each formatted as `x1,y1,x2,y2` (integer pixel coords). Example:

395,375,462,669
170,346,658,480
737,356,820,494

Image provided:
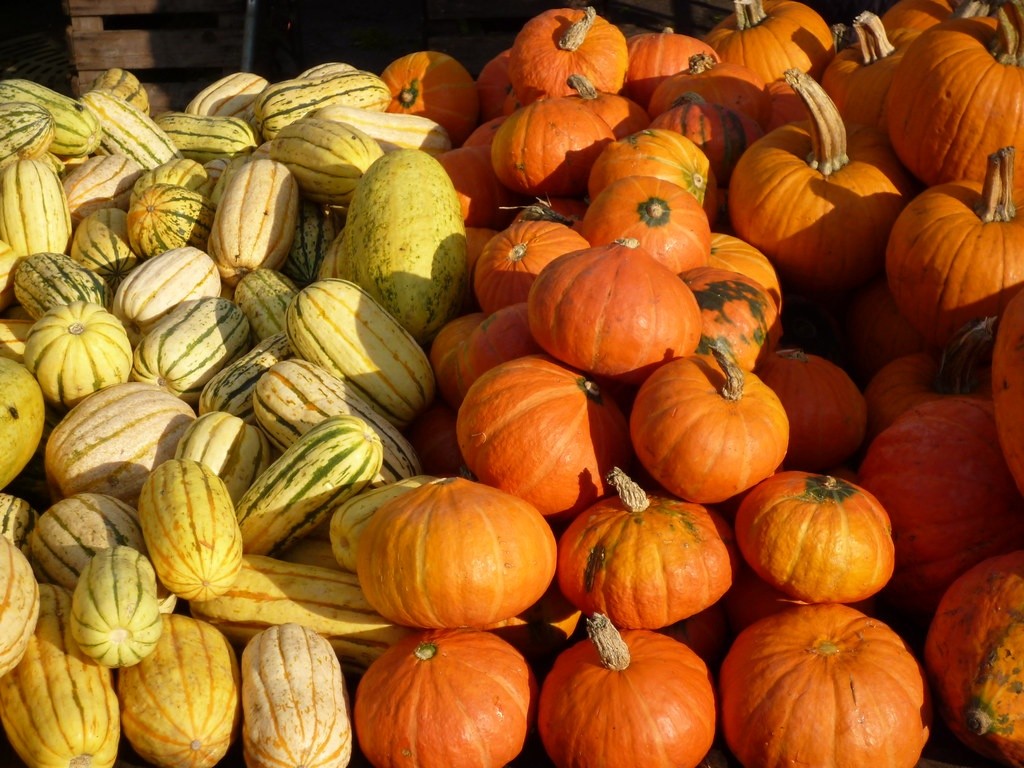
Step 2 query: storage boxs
425,0,734,79
59,0,305,107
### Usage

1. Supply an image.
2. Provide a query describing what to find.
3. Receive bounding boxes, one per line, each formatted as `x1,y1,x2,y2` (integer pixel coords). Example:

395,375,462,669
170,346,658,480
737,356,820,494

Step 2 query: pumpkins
0,60,464,768
353,0,1024,768
